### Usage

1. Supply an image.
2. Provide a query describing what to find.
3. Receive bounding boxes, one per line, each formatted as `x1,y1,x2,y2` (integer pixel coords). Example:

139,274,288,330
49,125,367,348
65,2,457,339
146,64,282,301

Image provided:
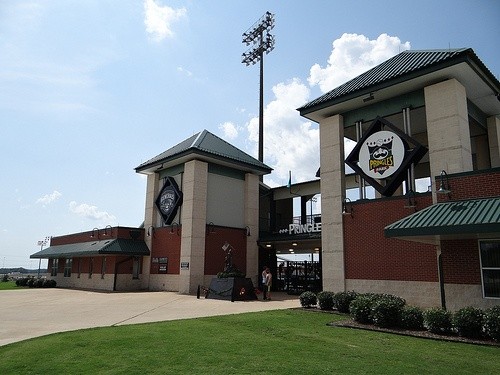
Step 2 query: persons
262,268,272,301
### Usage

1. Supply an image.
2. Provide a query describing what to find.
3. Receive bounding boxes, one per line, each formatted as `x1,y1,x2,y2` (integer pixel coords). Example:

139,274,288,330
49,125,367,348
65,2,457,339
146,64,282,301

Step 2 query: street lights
242,10,276,182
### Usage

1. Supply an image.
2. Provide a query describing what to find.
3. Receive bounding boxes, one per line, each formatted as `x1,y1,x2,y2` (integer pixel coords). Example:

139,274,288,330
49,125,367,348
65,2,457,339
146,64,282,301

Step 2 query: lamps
169,222,179,236
103,225,112,236
147,226,155,237
340,198,354,219
434,170,452,200
403,189,417,213
206,222,216,235
241,226,251,238
90,228,99,238
